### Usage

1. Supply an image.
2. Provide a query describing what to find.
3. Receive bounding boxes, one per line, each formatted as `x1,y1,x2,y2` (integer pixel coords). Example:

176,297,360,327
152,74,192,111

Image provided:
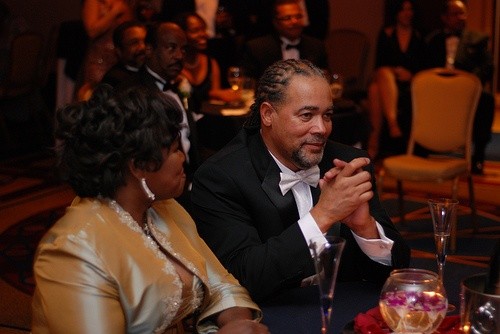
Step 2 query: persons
30,82,269,334
188,57,412,334
76,0,497,175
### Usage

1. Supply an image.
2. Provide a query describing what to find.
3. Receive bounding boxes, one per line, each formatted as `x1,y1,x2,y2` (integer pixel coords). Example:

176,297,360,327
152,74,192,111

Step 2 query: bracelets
446,57,455,65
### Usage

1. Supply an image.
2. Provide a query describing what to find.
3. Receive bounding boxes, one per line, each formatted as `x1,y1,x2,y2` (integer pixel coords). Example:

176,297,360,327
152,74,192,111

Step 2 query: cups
379,268,448,334
460,273,500,334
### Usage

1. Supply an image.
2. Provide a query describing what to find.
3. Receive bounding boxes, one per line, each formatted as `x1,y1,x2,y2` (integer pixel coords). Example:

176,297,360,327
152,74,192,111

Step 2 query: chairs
0,30,54,131
376,67,482,256
0,184,78,334
325,29,369,107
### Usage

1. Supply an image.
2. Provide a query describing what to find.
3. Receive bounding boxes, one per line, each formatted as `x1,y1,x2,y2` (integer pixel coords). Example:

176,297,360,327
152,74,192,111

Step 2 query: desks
201,87,367,119
261,237,381,334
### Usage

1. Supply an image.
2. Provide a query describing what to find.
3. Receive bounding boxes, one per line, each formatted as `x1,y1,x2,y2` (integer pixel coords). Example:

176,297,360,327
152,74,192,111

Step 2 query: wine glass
427,198,459,314
310,236,346,334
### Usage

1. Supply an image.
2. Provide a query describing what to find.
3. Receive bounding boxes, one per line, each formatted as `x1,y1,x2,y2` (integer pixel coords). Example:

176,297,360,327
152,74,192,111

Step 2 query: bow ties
163,82,178,92
278,164,320,196
286,43,299,49
